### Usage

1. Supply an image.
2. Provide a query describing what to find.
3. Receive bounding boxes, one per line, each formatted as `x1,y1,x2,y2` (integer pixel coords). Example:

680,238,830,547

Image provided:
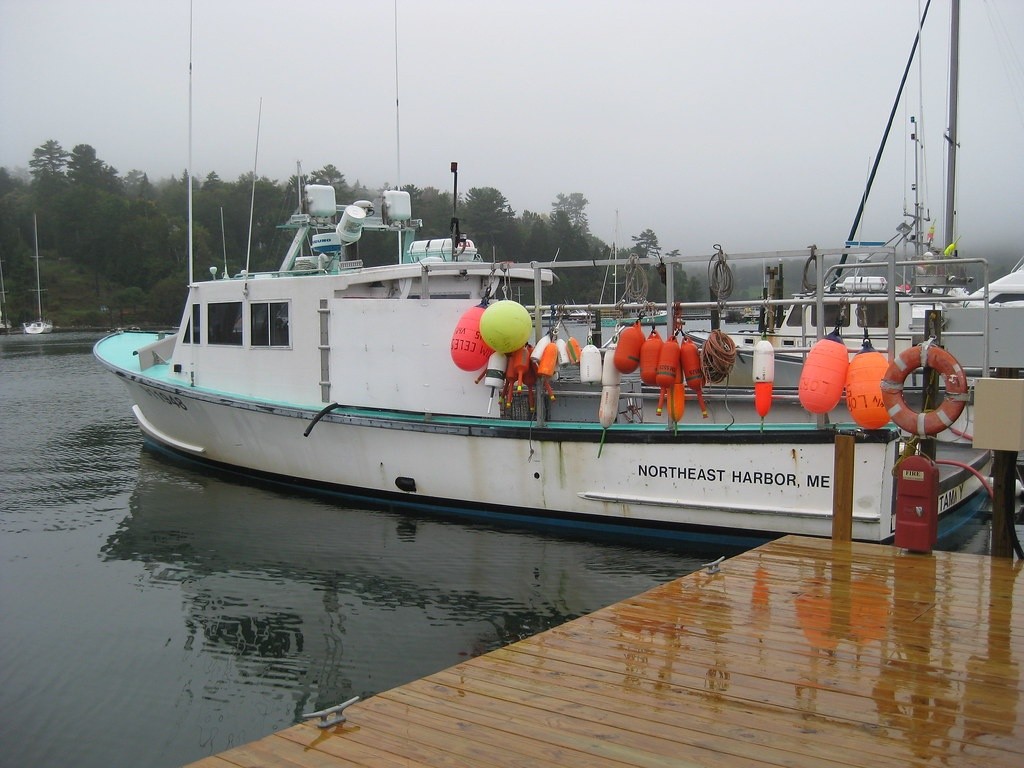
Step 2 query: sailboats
0,259,12,334
21,212,56,334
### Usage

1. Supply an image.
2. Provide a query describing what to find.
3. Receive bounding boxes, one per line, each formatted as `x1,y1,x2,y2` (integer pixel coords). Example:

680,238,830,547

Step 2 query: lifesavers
880,344,968,437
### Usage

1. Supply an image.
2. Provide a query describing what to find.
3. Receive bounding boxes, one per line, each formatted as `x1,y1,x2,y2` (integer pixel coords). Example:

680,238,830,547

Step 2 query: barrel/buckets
336,205,366,242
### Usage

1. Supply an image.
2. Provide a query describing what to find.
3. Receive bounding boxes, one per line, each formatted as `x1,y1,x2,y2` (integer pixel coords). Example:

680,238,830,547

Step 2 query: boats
89,0,1023,551
